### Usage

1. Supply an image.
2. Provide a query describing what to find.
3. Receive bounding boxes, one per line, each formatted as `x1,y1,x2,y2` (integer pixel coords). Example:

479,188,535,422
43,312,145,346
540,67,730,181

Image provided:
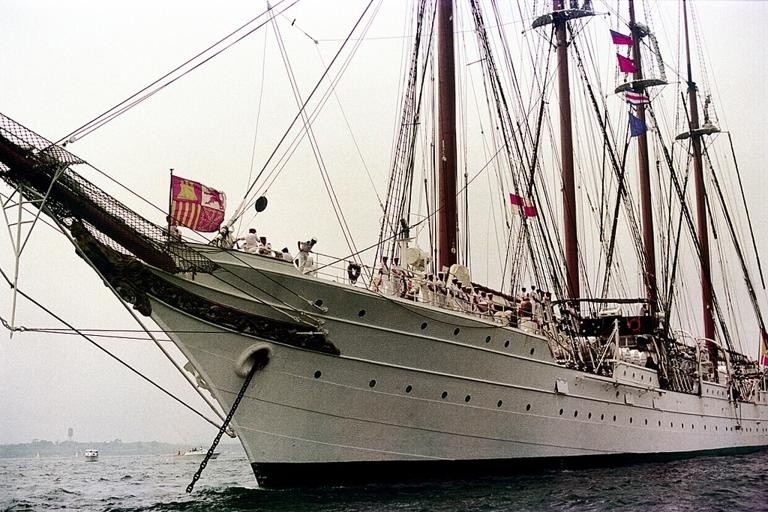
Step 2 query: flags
524,206,536,217
510,192,524,206
170,168,226,232
610,28,652,138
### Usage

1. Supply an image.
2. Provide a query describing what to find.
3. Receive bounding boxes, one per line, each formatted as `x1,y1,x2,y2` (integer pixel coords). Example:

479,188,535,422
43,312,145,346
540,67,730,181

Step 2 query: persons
161,215,175,235
377,254,553,332
258,236,271,255
293,239,317,268
282,247,291,259
243,228,259,253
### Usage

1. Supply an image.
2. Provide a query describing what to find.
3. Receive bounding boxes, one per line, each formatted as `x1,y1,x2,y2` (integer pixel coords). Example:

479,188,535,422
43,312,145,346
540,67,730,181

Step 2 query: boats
183,446,220,462
82,448,100,464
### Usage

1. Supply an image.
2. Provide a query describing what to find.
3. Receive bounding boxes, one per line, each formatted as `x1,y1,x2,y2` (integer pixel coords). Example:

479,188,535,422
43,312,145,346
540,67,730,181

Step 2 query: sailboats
0,1,767,512
